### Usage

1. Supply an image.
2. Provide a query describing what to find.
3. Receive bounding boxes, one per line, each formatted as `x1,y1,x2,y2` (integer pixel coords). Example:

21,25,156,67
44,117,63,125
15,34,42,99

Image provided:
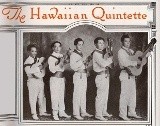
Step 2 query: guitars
125,38,156,76
105,37,114,73
55,48,71,78
31,49,52,79
79,49,96,69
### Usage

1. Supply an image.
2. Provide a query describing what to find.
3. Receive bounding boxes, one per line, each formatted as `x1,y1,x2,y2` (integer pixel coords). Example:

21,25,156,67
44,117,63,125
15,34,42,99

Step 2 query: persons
91,37,114,120
24,45,51,120
47,41,72,121
117,35,144,122
69,37,94,120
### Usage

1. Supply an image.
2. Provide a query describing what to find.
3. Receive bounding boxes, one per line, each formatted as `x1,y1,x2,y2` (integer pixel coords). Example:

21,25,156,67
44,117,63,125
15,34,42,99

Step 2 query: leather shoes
128,114,142,119
118,116,131,121
74,115,84,120
103,114,113,117
94,116,108,121
59,112,71,118
39,112,52,116
81,113,94,117
53,111,59,121
32,114,39,120
